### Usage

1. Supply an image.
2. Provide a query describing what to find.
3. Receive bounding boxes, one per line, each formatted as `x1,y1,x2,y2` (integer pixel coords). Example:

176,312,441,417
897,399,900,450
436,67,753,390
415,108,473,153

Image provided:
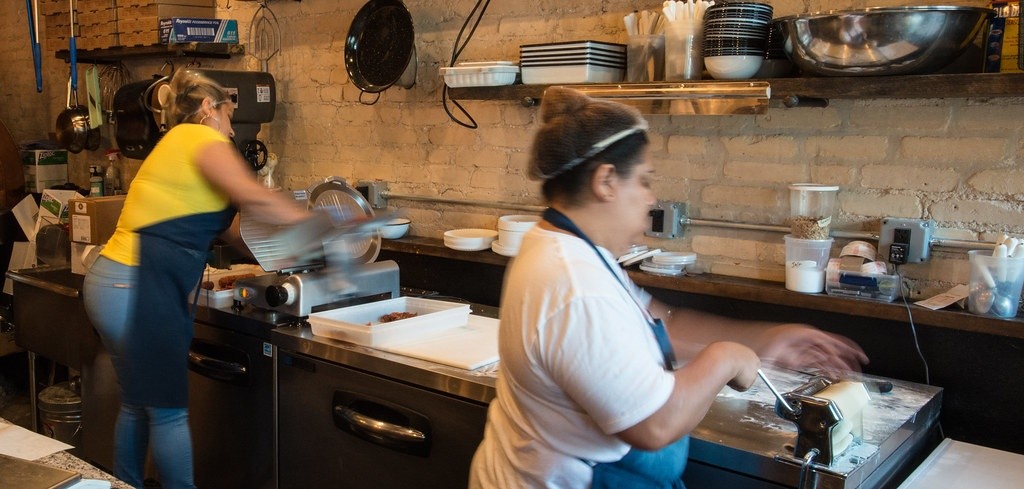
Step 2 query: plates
491,240,522,256
444,228,497,251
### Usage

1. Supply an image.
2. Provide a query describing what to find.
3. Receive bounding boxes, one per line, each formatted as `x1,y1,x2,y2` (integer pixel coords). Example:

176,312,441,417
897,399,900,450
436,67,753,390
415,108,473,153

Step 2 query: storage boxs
11,149,127,275
825,268,902,304
40,0,240,52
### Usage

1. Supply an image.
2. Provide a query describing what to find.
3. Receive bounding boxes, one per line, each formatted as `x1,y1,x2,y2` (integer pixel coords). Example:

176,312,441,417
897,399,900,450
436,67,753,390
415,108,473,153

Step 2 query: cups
497,215,543,250
788,182,839,238
626,19,703,82
966,250,1024,318
784,234,834,294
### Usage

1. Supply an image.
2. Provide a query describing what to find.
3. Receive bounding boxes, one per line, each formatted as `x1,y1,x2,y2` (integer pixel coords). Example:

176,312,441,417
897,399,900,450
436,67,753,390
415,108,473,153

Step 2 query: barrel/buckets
38,385,85,460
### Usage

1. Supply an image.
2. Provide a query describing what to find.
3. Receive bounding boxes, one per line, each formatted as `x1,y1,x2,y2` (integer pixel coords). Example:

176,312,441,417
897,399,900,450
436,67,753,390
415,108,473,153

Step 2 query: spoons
970,231,1024,319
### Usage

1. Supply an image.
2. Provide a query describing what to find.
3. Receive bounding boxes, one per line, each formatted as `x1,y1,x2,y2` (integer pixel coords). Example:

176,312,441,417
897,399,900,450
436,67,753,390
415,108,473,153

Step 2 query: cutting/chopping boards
379,313,520,372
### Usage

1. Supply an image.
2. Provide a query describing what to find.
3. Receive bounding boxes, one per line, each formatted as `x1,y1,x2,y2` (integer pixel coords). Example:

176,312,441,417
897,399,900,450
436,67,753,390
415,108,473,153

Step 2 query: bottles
88,153,123,197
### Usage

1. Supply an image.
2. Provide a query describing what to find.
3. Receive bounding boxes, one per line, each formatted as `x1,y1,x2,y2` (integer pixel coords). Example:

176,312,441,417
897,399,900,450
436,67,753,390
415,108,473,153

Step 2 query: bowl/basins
768,5,996,77
825,238,902,302
701,2,773,78
378,218,411,240
439,40,627,88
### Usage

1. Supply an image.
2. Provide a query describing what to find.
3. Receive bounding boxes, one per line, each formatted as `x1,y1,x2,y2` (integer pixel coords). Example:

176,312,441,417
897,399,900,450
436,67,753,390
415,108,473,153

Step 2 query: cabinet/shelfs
380,73,1024,340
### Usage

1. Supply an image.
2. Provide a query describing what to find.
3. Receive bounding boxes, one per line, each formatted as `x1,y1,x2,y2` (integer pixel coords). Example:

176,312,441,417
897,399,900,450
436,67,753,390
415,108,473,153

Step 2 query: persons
82,71,318,489
469,86,868,489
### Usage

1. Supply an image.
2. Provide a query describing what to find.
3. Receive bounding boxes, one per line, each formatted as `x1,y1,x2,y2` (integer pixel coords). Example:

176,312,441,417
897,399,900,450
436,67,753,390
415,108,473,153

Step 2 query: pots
56,64,100,154
344,0,415,105
